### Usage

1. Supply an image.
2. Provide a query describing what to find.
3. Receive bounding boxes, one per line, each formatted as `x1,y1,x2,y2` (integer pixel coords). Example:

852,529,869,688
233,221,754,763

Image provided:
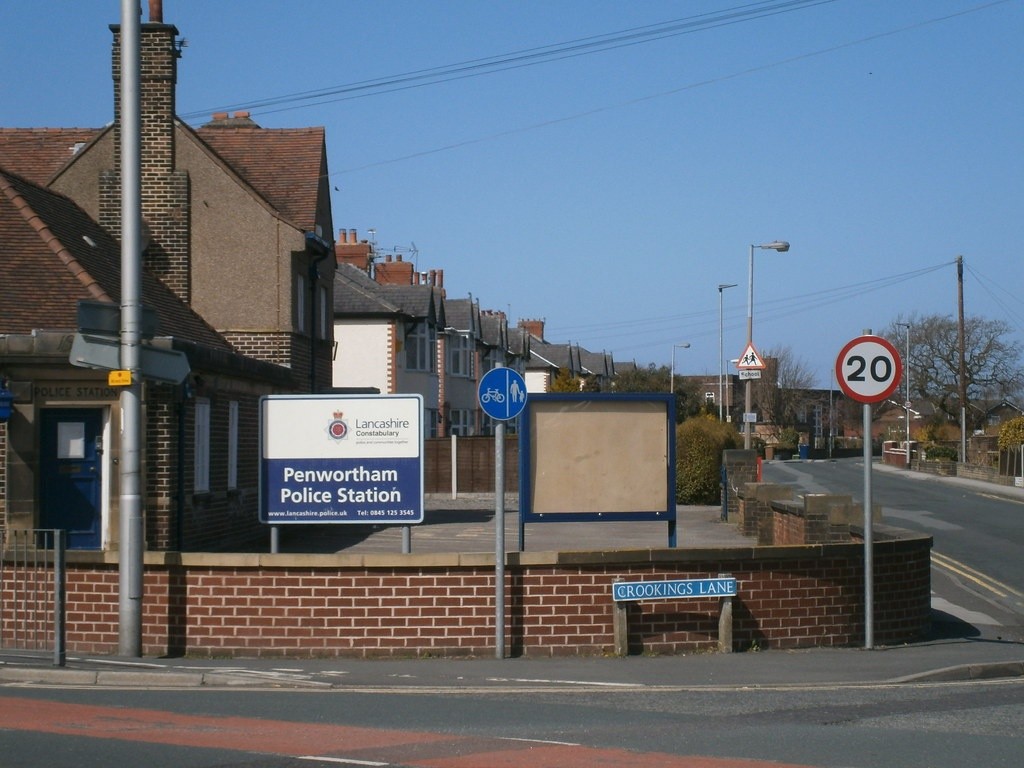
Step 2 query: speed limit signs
836,334,903,403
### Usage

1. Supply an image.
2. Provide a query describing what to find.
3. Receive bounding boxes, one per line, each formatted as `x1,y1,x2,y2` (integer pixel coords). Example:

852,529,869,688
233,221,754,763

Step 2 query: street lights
897,323,911,469
741,239,792,449
718,283,738,424
671,342,691,394
726,359,740,424
